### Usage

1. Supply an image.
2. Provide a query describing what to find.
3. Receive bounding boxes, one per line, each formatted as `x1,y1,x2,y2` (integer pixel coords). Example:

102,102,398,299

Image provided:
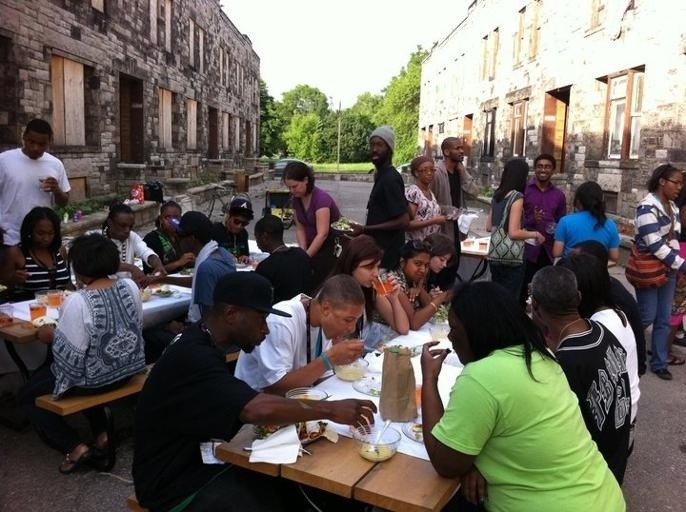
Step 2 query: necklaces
30,246,57,274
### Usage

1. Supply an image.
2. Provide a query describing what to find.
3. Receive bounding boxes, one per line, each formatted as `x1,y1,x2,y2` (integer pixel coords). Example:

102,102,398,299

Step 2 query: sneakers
656,368,672,380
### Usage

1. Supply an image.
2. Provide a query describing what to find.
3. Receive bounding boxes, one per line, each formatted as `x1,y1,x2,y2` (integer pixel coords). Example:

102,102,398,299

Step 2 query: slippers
668,354,685,365
59,440,108,474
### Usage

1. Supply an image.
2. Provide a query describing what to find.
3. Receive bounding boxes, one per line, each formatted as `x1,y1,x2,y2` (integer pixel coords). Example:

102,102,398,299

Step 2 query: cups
169,216,182,231
416,383,422,409
373,273,394,297
139,278,175,302
39,175,53,191
463,235,488,251
0,290,66,327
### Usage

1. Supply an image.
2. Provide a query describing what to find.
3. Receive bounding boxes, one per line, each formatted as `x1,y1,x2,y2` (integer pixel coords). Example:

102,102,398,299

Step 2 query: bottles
63,209,81,223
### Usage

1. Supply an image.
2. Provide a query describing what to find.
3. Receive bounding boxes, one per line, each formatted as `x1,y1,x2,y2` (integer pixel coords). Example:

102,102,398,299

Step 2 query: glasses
49,269,57,291
664,177,685,188
408,240,433,253
232,216,249,226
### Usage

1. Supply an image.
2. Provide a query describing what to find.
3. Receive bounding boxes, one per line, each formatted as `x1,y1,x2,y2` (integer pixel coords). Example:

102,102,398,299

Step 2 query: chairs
263,189,295,230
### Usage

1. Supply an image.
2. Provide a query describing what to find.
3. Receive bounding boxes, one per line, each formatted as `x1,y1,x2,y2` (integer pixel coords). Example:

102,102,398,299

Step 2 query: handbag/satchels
625,239,673,291
486,225,525,268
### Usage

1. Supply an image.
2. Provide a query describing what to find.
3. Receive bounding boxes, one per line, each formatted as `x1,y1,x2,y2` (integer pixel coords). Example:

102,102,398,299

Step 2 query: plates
254,422,327,445
401,418,424,443
351,374,382,396
330,220,358,233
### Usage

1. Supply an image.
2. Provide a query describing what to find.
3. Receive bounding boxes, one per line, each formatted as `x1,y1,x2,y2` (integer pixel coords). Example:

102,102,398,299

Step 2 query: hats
229,199,254,214
213,271,292,317
168,211,213,234
369,125,395,154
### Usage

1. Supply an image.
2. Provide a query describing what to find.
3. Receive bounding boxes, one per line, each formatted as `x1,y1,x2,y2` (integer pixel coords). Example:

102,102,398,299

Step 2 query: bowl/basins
351,424,402,463
284,355,369,401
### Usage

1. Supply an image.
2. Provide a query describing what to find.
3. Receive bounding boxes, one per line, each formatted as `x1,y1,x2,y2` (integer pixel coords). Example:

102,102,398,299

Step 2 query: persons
633,162,686,381
30,232,150,475
0,116,72,302
0,204,76,306
647,167,686,368
77,127,646,512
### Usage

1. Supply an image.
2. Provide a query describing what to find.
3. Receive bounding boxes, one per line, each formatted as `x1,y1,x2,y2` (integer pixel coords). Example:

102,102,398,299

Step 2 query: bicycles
204,182,249,222
